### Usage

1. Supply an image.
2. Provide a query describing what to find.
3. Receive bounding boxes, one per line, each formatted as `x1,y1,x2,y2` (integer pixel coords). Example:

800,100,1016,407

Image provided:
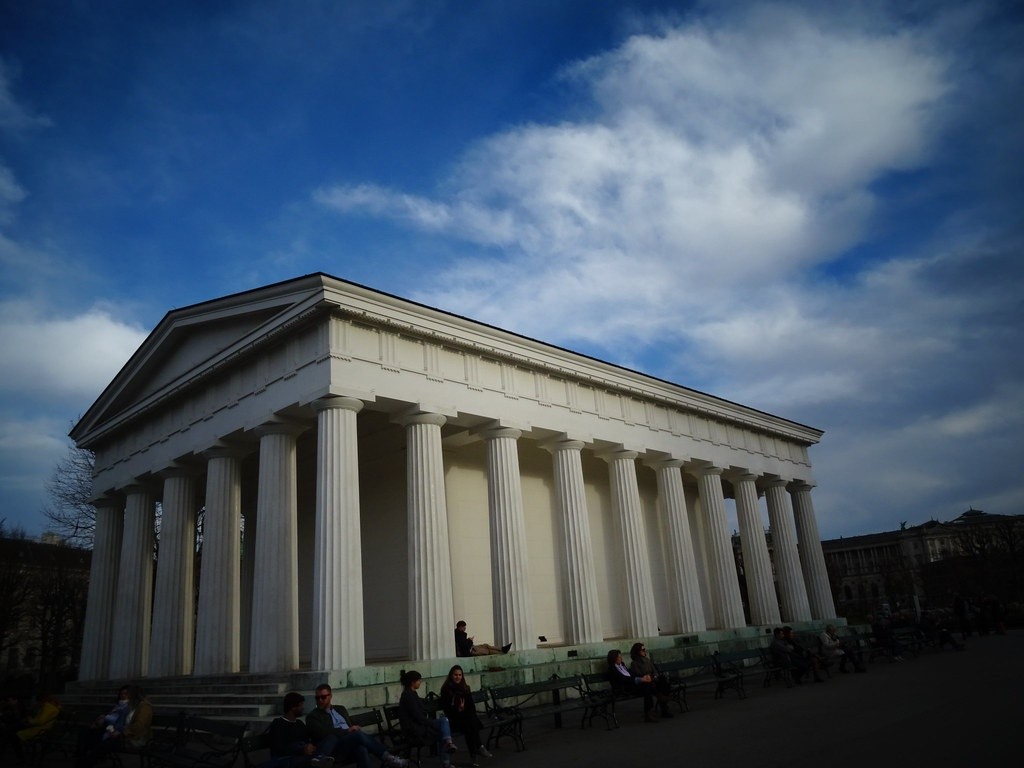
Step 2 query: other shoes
440,763,456,768
813,672,824,682
445,743,459,752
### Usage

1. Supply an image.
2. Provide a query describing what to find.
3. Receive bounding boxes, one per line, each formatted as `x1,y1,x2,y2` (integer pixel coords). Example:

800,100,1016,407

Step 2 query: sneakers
647,710,659,723
387,755,408,767
502,643,512,653
311,753,334,766
471,759,480,767
478,745,495,760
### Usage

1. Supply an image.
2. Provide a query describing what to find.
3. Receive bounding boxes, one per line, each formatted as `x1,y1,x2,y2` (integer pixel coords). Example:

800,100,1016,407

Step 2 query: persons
269,685,408,768
820,624,865,673
770,626,824,683
603,643,675,722
0,694,63,765
440,666,495,768
455,621,511,657
400,670,458,768
74,684,153,768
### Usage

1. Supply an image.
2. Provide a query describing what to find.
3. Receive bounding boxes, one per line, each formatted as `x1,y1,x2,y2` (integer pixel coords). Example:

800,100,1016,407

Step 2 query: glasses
315,693,332,700
641,648,646,652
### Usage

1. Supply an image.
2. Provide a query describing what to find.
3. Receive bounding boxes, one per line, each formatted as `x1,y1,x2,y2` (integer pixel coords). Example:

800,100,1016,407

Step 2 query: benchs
36,710,117,768
759,640,866,687
580,663,686,729
893,625,944,657
660,655,745,712
707,648,791,699
239,709,395,768
383,689,522,768
840,633,919,670
146,715,251,768
488,674,613,752
111,716,179,768
